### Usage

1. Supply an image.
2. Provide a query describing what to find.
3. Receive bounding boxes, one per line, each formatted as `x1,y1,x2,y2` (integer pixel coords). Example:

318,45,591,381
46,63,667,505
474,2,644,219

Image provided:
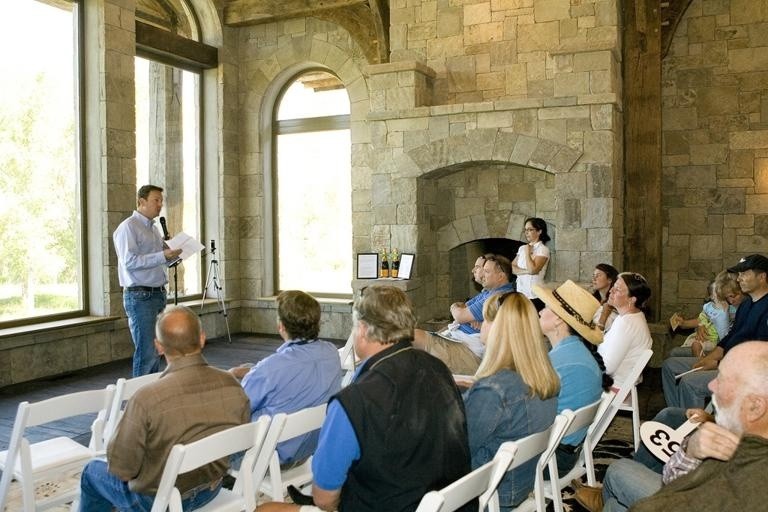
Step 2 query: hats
530,278,605,346
727,254,768,274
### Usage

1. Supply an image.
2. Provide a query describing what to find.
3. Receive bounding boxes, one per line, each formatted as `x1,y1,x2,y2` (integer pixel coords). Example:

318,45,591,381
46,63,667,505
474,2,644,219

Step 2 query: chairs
578,347,657,484
412,450,515,510
538,398,607,511
335,330,362,392
94,372,171,457
227,402,334,501
148,414,273,510
485,414,566,510
1,384,116,510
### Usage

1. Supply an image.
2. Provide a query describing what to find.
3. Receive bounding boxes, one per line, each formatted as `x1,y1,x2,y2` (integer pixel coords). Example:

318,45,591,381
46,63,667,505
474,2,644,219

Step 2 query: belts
126,284,166,292
181,476,224,502
558,443,586,454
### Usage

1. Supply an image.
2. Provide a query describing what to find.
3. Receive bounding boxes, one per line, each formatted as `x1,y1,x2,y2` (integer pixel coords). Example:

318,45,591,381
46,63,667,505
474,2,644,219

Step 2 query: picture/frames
356,252,379,280
398,252,417,281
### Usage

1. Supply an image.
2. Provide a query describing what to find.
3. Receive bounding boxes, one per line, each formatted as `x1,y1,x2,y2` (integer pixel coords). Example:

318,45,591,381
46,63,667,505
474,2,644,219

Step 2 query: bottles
380,246,390,278
392,248,399,279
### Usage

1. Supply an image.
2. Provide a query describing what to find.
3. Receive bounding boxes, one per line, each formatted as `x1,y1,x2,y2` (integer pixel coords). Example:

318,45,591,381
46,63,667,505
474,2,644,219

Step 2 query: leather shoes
571,479,605,512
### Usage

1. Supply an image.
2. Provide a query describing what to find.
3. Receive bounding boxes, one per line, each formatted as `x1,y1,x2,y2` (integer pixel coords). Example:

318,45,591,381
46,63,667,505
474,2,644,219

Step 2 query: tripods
200,259,232,344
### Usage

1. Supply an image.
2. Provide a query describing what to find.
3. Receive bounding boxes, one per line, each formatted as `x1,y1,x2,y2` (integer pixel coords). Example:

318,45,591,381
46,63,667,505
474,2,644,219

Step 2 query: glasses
489,256,504,272
525,229,536,231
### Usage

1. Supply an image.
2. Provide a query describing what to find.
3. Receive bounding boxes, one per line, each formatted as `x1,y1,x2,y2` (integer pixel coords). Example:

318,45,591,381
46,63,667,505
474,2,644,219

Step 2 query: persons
412,253,768,512
79,304,250,512
252,283,473,512
113,185,184,378
511,218,551,316
222,291,342,490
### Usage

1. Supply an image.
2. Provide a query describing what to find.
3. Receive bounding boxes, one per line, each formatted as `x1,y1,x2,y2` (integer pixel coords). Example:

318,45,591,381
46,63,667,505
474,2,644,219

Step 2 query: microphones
159,217,169,239
